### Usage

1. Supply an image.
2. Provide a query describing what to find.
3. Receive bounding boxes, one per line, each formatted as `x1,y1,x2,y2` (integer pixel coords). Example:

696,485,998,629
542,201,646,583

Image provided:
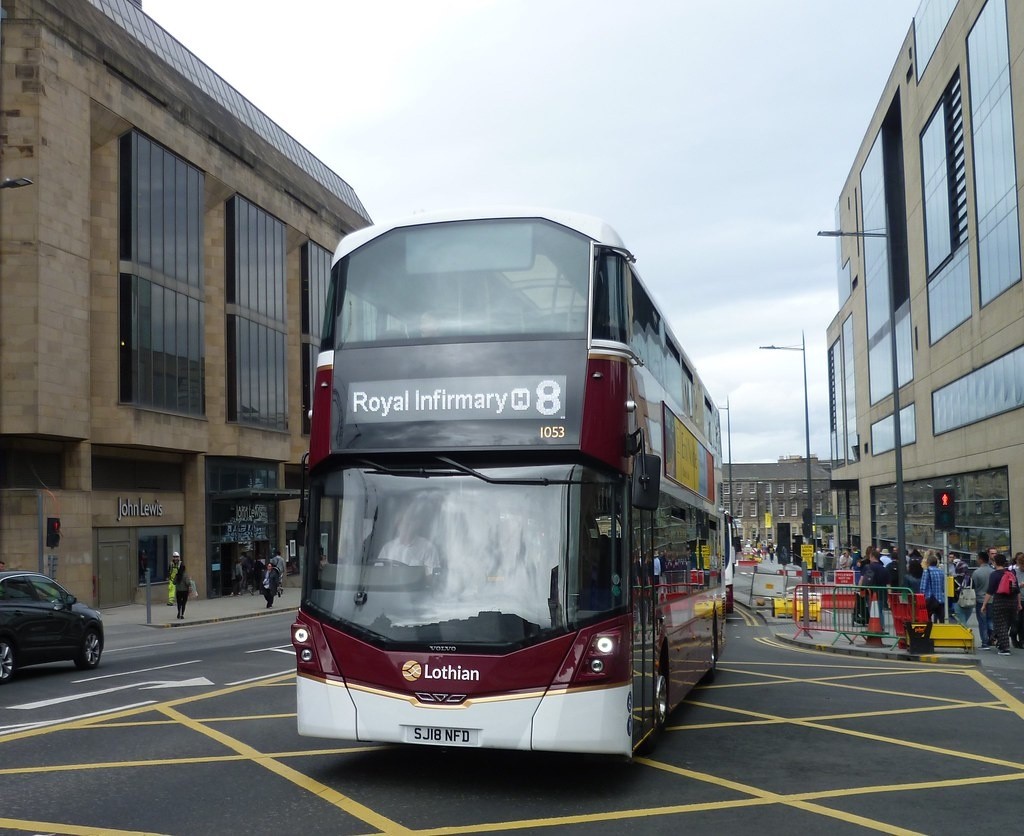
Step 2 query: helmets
173,552,180,557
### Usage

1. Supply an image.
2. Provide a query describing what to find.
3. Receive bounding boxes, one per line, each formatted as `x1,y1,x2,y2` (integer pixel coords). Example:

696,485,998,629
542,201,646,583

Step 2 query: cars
0,570,104,685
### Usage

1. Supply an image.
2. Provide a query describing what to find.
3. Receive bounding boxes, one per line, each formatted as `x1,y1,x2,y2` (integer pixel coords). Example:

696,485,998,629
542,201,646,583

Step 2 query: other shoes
229,592,237,596
977,644,991,650
181,615,184,619
177,615,180,619
277,591,282,598
237,593,244,597
997,649,1011,655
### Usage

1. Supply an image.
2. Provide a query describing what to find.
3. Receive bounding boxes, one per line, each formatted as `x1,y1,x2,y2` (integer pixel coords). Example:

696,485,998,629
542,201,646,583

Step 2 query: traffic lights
46,517,61,547
934,488,957,530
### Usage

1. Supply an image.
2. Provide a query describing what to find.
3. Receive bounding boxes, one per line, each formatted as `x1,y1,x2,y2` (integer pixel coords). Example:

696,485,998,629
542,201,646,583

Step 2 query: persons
636,550,687,594
375,515,442,585
166,552,183,606
173,565,192,619
0,561,5,572
757,544,1024,655
230,551,286,609
139,549,149,581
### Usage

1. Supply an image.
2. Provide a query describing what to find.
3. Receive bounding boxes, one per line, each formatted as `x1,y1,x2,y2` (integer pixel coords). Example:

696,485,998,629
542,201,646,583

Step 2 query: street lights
817,205,905,589
759,327,814,546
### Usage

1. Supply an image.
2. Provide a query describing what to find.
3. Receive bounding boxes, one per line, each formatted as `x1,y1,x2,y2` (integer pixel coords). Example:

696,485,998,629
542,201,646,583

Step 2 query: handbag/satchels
188,580,198,599
262,579,269,589
235,574,242,583
924,593,941,615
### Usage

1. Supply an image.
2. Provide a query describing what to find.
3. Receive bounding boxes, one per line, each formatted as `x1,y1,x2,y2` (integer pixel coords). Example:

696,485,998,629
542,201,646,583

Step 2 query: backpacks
996,570,1020,595
769,547,774,553
953,577,978,607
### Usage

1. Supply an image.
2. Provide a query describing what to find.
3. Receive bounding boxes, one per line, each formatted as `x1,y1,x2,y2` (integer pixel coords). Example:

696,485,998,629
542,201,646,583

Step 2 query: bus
723,509,742,615
291,202,716,759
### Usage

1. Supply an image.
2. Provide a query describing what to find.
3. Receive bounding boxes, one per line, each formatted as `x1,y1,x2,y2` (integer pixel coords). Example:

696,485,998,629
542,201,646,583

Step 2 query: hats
654,551,659,556
954,561,969,574
879,549,890,555
952,559,962,564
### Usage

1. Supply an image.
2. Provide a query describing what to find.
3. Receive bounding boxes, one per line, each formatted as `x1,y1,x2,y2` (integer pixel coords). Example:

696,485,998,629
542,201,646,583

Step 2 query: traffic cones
861,592,889,635
857,636,893,649
